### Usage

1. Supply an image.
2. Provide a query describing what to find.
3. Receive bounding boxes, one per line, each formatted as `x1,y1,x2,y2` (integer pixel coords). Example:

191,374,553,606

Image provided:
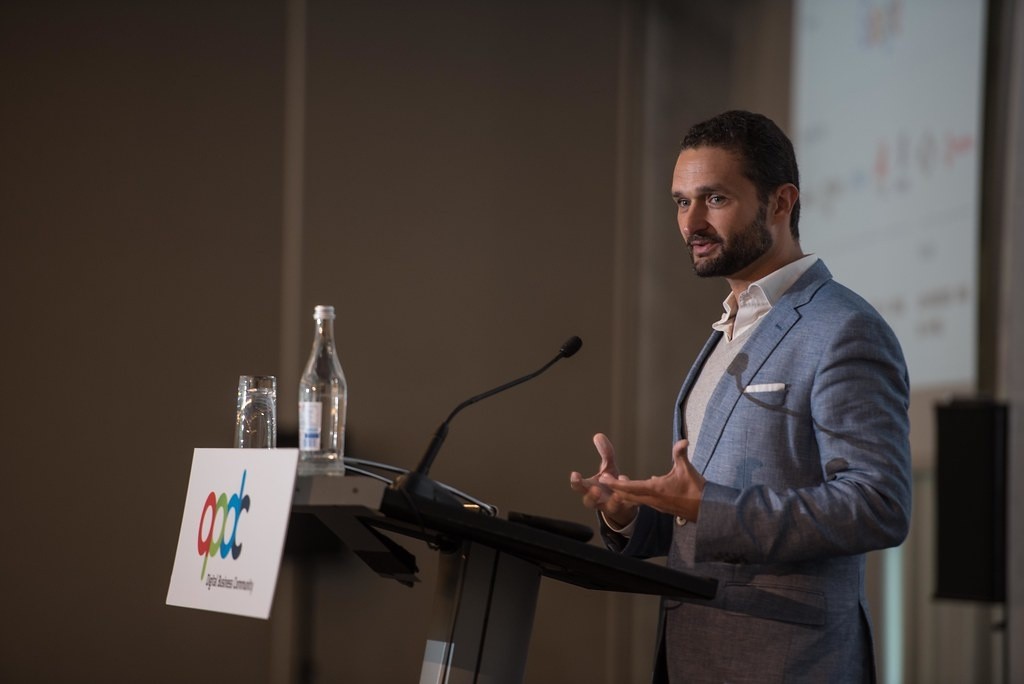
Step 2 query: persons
570,112,913,683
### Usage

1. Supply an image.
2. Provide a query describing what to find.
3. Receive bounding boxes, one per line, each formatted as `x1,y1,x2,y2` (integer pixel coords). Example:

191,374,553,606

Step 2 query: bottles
296,305,345,476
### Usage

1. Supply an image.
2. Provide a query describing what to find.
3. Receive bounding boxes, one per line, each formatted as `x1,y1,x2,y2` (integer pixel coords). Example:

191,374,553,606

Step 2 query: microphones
379,336,583,526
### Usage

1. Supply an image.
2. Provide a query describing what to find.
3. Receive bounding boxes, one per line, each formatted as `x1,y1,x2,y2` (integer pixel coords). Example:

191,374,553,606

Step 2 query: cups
234,375,276,449
